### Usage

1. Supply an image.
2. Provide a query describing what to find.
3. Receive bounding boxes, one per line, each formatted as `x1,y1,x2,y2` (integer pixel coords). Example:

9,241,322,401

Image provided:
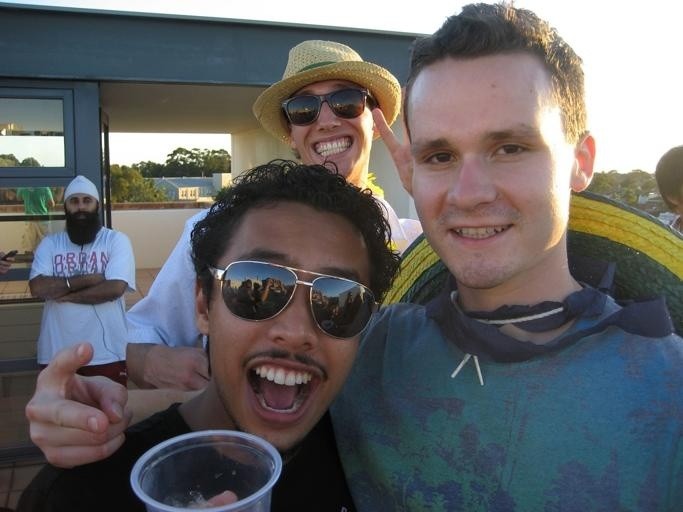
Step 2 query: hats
381,189,683,337
65,175,100,200
252,39,401,145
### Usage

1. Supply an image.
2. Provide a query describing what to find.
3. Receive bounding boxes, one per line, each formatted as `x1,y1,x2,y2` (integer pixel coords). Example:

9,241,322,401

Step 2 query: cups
129,430,283,512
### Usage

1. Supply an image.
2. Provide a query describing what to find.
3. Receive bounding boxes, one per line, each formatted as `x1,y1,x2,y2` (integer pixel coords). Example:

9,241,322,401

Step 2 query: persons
16,154,402,512
655,145,683,235
16,157,55,253
120,38,425,388
27,174,138,389
0,250,16,275
24,2,683,512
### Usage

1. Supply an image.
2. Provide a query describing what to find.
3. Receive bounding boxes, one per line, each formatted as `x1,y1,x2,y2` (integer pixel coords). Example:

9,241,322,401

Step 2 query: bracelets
65,276,74,292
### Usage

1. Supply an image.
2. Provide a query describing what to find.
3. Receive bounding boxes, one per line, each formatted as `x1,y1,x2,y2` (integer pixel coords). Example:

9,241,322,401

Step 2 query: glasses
282,87,376,126
209,261,381,337
